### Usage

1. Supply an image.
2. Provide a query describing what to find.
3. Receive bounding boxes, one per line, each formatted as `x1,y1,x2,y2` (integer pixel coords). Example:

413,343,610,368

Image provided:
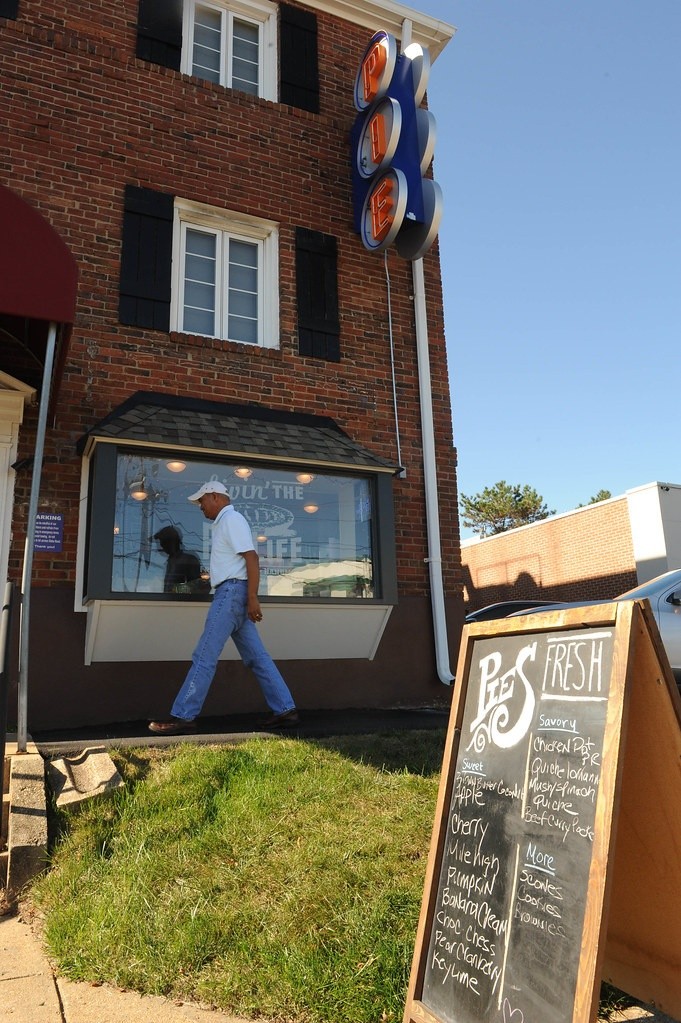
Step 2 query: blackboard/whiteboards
410,600,639,1023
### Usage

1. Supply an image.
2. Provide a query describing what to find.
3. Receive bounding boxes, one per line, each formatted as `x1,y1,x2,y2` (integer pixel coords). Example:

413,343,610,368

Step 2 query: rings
256,613,259,617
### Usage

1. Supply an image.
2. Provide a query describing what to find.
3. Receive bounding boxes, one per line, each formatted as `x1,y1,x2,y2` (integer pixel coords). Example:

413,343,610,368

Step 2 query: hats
152,525,182,541
187,481,229,506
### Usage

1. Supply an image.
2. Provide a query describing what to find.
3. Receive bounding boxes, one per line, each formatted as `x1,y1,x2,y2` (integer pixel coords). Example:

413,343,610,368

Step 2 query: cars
467,568,681,689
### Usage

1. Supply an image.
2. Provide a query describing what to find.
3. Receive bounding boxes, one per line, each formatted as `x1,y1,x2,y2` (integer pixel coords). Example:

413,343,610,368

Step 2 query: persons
149,481,302,734
153,524,210,594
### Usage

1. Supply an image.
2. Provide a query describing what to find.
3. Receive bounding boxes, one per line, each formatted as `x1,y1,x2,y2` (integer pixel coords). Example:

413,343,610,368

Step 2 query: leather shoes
148,717,196,735
263,711,298,730
